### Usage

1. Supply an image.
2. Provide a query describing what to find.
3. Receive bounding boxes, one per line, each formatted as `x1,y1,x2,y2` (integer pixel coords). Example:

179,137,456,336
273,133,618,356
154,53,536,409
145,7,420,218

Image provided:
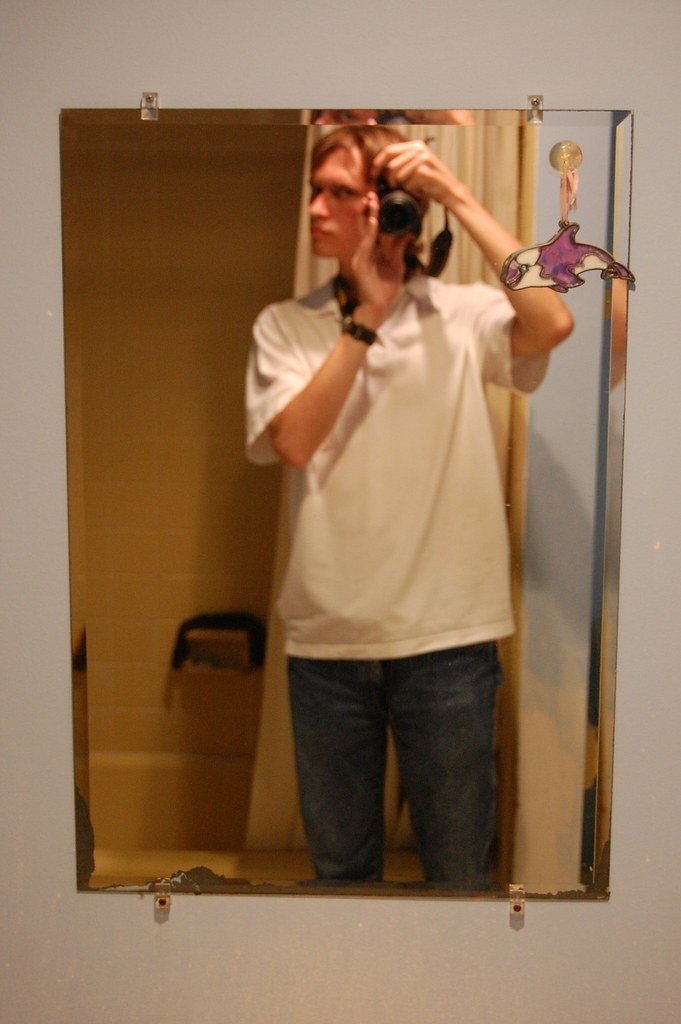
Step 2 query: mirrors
59,93,634,915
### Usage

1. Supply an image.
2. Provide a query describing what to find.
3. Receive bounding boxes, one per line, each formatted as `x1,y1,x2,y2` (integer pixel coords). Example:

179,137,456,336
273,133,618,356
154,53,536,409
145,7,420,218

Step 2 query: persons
245,123,575,889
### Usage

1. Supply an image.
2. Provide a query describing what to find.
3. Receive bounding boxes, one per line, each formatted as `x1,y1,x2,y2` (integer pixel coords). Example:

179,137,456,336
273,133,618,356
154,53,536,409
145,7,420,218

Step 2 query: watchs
341,316,376,345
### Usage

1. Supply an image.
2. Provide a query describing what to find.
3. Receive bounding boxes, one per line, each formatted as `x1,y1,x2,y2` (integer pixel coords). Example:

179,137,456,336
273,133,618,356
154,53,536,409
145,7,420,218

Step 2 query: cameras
376,172,422,240
